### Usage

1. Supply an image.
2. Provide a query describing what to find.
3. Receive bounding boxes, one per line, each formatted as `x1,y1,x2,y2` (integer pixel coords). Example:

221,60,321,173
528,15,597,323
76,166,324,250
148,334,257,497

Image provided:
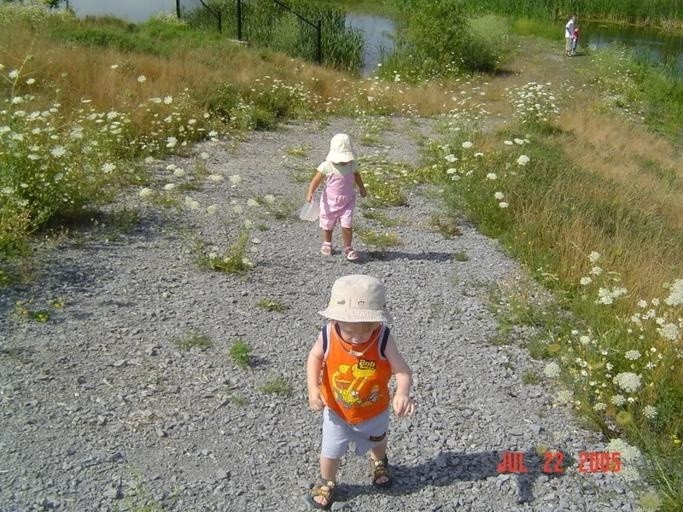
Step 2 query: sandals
320,240,332,255
343,245,359,260
307,475,337,509
371,454,392,486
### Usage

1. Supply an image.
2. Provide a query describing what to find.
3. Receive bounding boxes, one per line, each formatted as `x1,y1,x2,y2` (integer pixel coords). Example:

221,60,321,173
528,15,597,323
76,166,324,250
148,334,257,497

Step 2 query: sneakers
566,51,576,57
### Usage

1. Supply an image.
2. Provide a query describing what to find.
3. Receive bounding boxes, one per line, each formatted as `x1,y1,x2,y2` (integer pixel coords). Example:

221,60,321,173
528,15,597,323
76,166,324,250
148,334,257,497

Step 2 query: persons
564,13,581,57
305,133,367,261
307,274,414,509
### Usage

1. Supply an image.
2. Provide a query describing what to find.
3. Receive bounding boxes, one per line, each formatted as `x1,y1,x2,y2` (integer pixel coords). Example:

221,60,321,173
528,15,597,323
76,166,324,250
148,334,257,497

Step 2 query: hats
318,274,394,323
325,133,355,163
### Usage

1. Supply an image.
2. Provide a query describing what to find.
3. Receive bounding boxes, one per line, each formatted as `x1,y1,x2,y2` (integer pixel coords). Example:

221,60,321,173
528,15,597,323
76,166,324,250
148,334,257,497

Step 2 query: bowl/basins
300,201,320,221
